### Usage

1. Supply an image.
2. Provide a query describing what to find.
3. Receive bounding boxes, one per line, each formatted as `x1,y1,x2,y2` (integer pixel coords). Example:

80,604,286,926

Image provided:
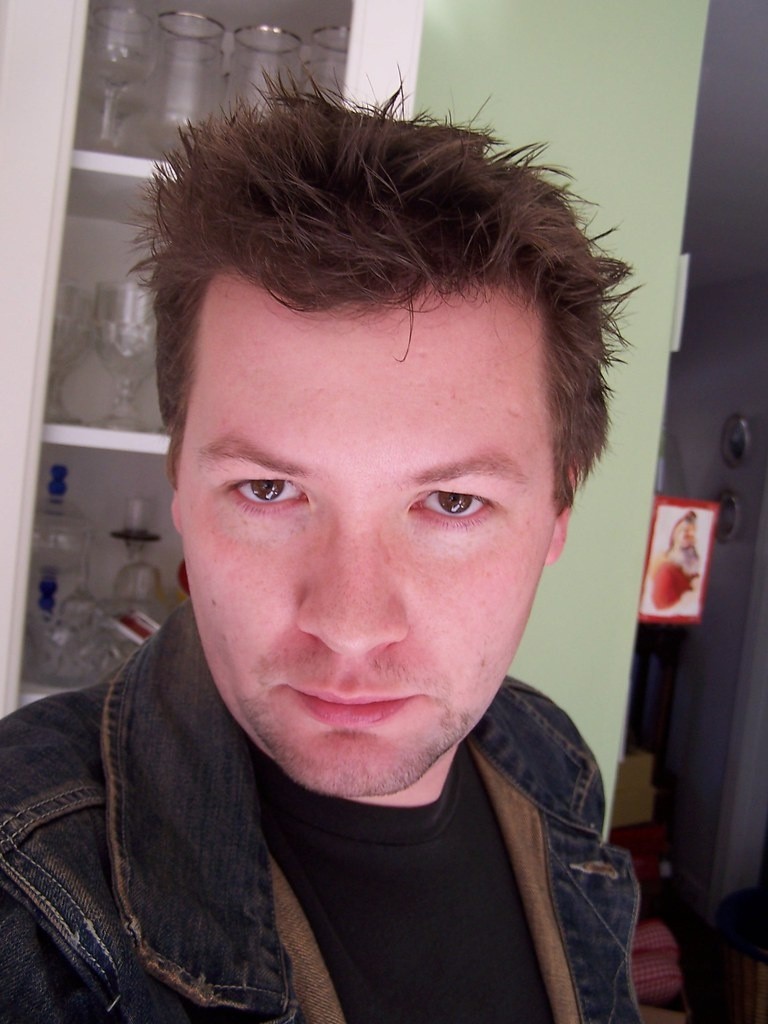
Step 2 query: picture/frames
637,496,719,625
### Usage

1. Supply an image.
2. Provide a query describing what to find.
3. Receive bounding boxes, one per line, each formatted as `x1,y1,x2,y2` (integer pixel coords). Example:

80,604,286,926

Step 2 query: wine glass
44,272,98,425
88,4,151,155
148,10,223,159
97,280,158,432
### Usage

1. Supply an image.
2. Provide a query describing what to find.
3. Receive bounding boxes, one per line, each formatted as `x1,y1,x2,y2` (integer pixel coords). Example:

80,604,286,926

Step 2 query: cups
225,25,304,117
311,25,347,104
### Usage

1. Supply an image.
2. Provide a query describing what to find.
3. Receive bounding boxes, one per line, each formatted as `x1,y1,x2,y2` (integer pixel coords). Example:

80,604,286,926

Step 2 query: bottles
20,464,91,686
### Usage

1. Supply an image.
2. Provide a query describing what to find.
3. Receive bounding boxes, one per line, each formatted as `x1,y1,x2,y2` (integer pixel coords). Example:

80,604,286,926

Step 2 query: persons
0,83,649,1024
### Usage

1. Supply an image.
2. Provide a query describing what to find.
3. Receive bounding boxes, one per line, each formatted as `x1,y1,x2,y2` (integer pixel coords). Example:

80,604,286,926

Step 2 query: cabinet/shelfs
626,625,693,759
0,0,425,727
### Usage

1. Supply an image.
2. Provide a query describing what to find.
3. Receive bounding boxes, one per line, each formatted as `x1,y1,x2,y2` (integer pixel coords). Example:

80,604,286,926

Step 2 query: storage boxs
630,854,661,881
716,886,768,1024
610,816,667,854
611,745,656,826
